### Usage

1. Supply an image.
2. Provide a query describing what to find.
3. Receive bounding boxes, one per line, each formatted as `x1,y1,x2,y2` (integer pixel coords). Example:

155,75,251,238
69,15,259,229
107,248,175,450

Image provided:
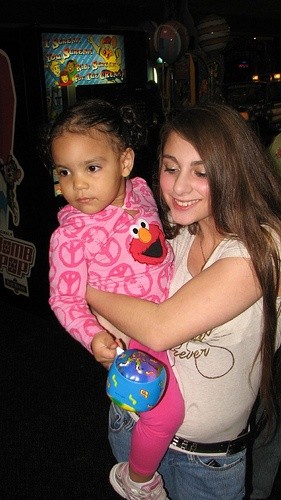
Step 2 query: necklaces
198,233,209,263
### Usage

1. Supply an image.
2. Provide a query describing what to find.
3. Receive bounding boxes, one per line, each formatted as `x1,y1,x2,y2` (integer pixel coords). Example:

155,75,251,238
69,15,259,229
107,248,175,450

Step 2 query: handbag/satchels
248,393,281,500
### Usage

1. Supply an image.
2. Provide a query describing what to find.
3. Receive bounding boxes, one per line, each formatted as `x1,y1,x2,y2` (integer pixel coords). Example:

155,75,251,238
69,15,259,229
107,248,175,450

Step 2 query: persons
48,99,185,500
85,103,281,500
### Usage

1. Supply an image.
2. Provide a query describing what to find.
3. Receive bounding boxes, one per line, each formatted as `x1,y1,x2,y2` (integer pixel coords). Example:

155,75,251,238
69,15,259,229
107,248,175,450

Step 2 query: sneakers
109,461,169,500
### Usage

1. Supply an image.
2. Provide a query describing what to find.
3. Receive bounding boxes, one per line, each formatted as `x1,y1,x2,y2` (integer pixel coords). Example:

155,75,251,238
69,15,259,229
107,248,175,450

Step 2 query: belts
170,432,249,457
